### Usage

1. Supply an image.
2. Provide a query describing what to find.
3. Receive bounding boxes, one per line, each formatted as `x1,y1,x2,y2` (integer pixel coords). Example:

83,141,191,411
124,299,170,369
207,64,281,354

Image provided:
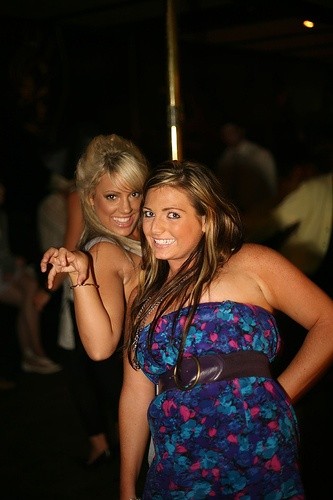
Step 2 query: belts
157,350,272,395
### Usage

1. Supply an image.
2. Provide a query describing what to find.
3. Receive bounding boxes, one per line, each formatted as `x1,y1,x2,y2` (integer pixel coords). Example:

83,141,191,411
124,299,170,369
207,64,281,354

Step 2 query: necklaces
132,268,193,369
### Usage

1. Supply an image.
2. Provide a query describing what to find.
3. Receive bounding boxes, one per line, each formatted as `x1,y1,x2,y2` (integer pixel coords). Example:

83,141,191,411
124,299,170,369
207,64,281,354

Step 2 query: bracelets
72,283,98,289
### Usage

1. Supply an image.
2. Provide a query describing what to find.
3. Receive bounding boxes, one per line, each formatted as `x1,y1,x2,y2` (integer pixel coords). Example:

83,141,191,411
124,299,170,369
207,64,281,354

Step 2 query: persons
156,42,333,500
119,161,333,500
41,134,149,500
0,59,121,469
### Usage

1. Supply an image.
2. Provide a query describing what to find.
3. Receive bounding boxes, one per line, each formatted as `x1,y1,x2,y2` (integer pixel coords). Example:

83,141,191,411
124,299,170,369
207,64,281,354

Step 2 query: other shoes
21,357,61,374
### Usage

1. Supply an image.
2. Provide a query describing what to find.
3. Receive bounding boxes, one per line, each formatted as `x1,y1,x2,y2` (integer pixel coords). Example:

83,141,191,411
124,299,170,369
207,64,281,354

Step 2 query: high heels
78,447,112,470
